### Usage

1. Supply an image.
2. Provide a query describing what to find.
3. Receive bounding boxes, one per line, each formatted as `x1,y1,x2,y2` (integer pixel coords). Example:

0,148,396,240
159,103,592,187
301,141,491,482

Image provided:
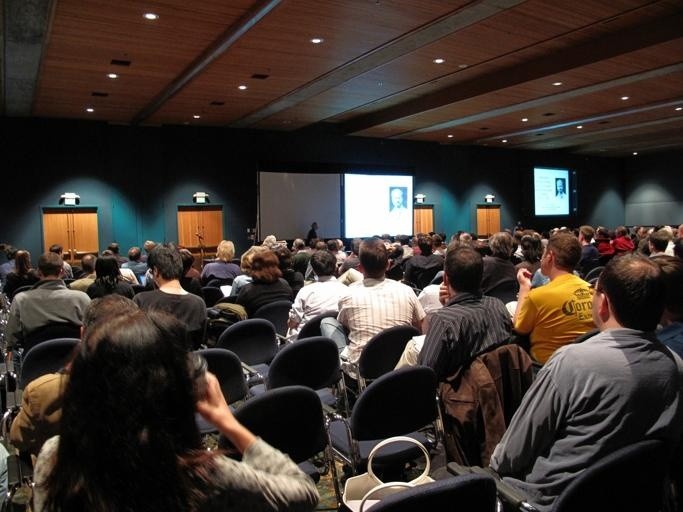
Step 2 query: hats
580,225,595,238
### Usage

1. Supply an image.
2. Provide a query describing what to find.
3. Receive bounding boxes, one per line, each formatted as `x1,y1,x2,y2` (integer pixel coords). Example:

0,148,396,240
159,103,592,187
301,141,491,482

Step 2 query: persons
556,179,566,198
391,188,407,211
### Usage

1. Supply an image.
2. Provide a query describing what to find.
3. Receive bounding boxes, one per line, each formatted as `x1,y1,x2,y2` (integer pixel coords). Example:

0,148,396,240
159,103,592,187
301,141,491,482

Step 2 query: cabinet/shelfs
177,211,223,248
476,208,500,237
43,213,99,255
413,208,433,237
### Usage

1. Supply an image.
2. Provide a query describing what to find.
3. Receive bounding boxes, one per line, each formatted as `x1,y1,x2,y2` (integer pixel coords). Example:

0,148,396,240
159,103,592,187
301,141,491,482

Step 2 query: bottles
184,355,209,409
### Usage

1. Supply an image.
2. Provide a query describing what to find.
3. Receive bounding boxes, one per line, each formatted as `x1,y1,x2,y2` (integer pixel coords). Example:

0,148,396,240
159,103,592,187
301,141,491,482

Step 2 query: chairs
1,266,667,512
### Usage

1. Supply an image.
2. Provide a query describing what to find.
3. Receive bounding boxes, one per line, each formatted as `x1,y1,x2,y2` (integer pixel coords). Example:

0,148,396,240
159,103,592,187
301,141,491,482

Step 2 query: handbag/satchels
339,435,435,511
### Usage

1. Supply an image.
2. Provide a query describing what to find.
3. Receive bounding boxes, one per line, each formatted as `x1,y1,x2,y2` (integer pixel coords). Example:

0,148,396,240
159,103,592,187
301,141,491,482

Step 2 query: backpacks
207,300,250,351
